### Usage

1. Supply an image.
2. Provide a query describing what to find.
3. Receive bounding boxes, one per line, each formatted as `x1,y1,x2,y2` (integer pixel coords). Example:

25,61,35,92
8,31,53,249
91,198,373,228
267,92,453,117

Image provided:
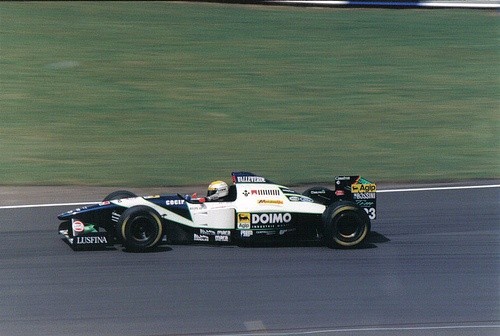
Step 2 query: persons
206,180,230,202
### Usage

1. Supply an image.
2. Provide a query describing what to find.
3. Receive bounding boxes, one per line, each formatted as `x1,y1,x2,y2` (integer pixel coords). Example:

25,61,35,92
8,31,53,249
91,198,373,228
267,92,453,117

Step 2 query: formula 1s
56,170,378,251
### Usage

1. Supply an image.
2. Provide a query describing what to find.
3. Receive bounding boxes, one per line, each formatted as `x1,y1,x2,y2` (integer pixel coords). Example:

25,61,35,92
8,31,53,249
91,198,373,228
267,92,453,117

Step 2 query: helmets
205,180,229,201
